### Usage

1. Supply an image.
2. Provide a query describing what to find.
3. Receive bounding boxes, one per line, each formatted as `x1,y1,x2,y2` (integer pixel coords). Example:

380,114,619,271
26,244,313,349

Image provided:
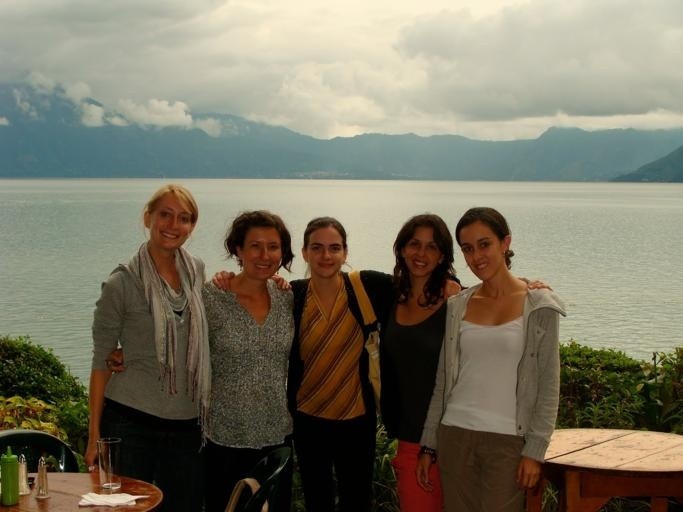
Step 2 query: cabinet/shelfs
0,446,47,506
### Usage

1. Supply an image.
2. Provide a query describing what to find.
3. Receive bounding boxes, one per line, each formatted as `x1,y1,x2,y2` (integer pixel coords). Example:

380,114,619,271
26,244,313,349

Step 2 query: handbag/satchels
417,446,437,464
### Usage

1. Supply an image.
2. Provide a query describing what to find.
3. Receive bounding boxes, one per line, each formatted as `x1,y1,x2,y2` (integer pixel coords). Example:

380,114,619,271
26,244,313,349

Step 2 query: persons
415,207,566,512
377,215,555,512
108,212,296,512
213,217,462,512
84,185,293,512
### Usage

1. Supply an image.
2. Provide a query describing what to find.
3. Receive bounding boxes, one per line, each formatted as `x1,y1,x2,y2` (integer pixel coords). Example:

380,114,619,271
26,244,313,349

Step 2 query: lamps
0,429,80,473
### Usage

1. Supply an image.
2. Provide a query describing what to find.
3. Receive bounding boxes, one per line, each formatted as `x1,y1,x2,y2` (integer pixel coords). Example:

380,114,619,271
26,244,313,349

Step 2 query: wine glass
87,465,98,472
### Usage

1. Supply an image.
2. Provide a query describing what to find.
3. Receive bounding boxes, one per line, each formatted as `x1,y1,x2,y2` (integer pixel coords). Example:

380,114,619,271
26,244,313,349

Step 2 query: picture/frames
524,428,683,512
0,473,165,512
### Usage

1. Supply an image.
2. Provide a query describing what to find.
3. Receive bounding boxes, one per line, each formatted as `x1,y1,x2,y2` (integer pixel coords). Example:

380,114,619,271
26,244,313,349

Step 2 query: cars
96,437,122,491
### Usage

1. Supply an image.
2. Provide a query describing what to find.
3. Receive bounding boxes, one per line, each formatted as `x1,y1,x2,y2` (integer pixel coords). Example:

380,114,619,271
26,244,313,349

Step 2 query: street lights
363,330,382,413
